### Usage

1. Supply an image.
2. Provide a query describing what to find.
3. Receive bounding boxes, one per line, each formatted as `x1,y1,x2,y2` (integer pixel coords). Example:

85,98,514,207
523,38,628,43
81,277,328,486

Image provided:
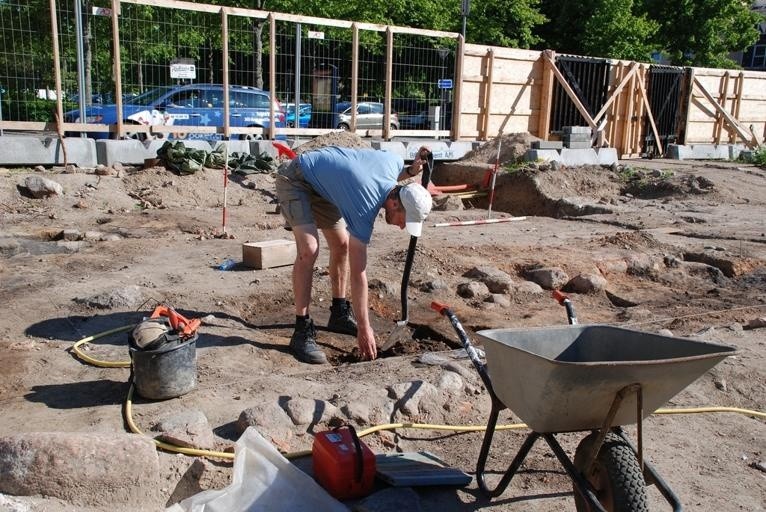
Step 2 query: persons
275,143,436,365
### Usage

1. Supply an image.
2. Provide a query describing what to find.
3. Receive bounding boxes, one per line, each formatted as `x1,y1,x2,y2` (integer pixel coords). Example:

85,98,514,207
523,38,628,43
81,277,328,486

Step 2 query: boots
288,319,327,364
327,300,358,335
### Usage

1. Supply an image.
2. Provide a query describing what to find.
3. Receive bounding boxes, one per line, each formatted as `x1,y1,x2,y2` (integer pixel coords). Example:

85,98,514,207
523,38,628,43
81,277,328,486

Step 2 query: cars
272,100,312,127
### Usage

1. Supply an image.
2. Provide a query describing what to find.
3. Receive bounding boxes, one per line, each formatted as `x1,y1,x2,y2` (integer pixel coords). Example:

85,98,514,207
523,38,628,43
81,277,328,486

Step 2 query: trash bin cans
427,103,443,132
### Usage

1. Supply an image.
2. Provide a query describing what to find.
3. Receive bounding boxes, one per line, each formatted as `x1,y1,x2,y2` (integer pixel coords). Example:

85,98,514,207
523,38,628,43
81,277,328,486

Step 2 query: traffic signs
439,80,452,88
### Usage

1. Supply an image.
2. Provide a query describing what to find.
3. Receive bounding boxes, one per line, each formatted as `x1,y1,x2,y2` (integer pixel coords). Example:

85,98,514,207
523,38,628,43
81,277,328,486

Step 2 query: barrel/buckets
130,334,200,400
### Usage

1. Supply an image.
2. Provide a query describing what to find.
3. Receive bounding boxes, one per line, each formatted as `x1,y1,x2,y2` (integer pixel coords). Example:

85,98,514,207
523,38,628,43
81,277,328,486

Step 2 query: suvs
335,99,401,132
62,79,286,142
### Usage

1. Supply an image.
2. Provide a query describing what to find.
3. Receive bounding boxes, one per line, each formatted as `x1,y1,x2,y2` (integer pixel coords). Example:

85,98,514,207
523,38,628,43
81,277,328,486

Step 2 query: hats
399,183,432,237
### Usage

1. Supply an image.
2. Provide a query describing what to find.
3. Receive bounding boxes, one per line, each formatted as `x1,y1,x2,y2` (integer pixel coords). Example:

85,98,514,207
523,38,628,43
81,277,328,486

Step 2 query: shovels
380,152,434,352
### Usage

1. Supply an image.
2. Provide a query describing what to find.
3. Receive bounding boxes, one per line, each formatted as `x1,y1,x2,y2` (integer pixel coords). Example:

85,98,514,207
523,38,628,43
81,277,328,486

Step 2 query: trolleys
430,287,741,511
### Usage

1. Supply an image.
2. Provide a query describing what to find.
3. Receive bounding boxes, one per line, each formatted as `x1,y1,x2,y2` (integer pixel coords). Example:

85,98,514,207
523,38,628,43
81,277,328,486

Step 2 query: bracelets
406,167,416,178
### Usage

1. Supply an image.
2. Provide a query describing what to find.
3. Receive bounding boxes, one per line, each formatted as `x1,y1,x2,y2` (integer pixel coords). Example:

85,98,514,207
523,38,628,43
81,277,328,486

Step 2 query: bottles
217,258,236,270
